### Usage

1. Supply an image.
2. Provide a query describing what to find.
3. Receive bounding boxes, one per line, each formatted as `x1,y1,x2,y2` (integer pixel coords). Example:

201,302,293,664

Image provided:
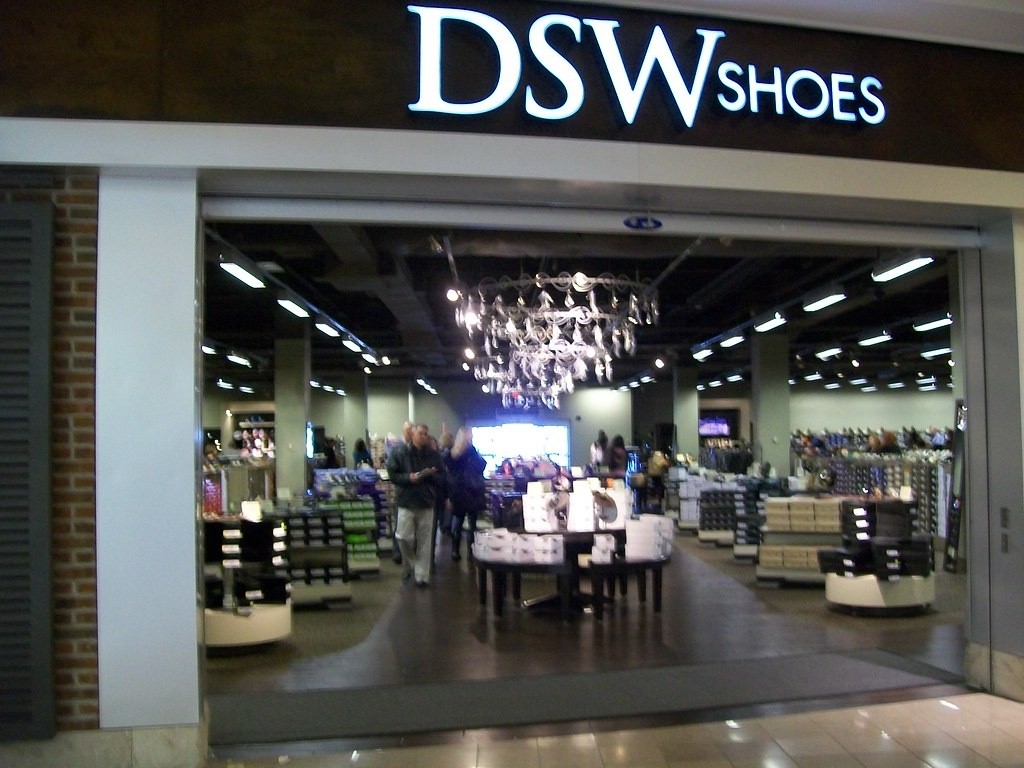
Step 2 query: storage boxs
201,471,935,606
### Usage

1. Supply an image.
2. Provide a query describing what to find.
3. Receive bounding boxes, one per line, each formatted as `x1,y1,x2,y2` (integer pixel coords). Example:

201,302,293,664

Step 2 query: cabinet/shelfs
699,486,740,548
280,508,353,609
733,488,802,564
313,496,381,576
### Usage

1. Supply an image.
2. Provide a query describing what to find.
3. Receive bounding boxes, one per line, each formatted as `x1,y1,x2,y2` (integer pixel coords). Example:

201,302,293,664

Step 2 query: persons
386,421,487,586
868,431,927,455
590,428,654,482
352,437,381,539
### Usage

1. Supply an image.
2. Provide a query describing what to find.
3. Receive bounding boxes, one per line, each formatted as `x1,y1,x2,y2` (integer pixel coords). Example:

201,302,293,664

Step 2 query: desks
468,528,675,623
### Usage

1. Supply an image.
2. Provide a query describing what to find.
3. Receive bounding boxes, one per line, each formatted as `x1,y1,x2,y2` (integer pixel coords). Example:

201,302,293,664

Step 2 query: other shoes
416,581,429,588
401,569,414,584
451,552,461,560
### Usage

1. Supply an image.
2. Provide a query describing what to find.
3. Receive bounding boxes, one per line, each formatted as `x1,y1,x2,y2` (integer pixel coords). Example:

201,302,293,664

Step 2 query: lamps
276,293,311,319
871,251,938,282
427,235,444,256
441,233,700,412
342,336,364,353
754,310,788,333
361,351,377,363
721,331,746,348
219,255,267,289
693,345,714,361
803,288,848,311
315,319,341,337
